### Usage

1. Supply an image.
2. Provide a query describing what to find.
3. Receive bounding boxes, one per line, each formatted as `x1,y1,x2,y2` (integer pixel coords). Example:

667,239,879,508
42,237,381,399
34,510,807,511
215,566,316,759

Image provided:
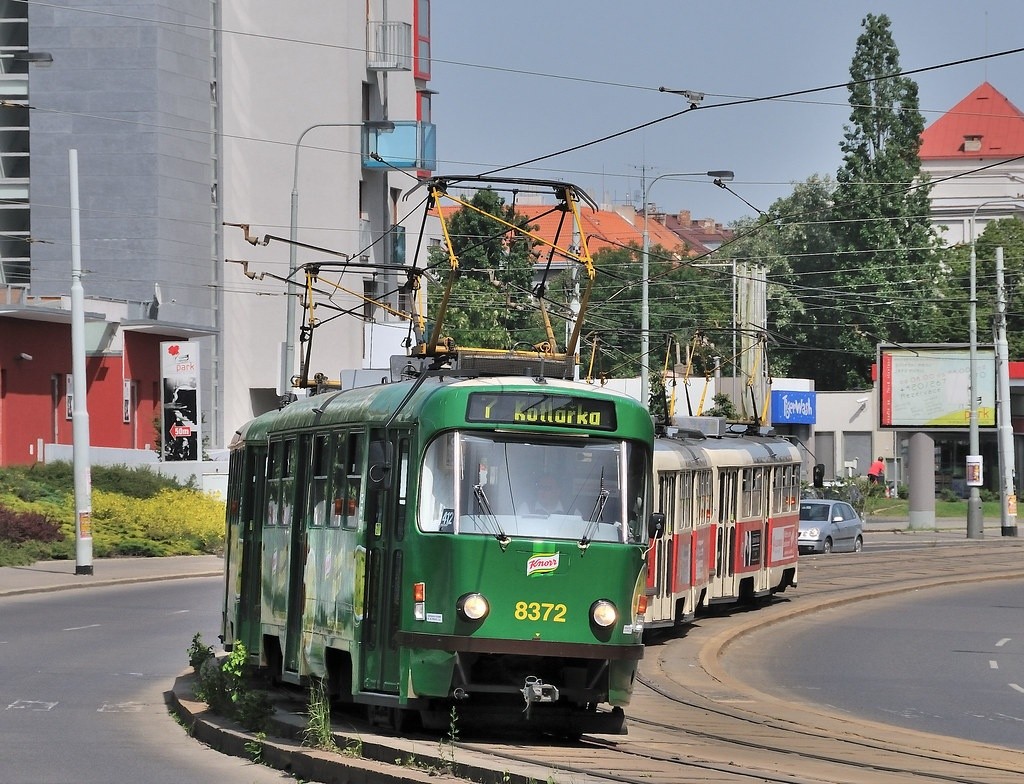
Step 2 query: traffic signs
170,422,193,441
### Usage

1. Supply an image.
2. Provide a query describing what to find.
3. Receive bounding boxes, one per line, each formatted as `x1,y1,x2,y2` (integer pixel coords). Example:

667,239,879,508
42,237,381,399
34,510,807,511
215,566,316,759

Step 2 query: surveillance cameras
856,398,868,403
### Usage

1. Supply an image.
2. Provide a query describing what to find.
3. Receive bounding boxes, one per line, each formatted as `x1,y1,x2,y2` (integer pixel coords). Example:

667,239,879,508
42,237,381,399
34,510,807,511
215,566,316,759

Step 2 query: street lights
639,169,737,407
282,118,400,395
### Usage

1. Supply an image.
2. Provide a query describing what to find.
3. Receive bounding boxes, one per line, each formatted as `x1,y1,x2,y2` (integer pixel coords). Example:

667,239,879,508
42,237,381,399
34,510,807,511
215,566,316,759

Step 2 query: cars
799,498,863,557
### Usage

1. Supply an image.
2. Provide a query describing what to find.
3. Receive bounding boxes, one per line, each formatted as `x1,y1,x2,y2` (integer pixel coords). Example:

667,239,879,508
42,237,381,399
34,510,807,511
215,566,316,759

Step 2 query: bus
220,171,665,741
566,319,802,645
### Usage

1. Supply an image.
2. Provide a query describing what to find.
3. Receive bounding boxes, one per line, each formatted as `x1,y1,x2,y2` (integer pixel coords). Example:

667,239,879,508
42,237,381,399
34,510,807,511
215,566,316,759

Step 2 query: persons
516,474,584,517
867,456,886,495
